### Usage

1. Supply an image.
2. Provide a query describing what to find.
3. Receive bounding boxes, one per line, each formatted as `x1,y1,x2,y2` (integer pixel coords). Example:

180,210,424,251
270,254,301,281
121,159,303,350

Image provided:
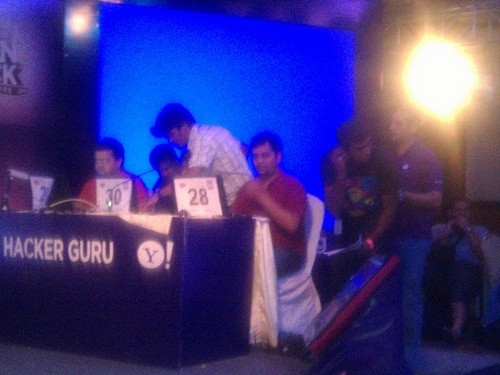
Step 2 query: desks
0,207,265,373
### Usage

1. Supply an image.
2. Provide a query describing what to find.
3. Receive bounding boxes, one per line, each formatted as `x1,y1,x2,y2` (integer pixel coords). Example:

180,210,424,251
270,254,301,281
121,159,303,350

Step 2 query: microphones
108,167,157,211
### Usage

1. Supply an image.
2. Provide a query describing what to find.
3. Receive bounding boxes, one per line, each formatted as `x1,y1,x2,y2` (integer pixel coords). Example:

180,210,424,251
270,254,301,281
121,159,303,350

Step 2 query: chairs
276,192,325,353
430,222,488,323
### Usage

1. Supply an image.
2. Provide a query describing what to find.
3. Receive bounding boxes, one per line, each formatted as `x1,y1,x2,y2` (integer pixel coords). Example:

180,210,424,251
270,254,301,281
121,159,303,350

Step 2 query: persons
73,136,152,214
424,197,489,341
146,142,184,214
226,133,309,279
370,103,443,352
144,100,253,216
320,117,402,290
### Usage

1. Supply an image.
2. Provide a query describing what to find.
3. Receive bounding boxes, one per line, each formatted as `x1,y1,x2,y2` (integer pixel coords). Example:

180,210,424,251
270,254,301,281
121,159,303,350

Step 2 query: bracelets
366,238,375,250
155,188,164,202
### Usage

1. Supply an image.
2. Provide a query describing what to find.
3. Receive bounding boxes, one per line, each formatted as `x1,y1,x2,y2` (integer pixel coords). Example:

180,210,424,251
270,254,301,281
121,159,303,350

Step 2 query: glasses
94,158,116,164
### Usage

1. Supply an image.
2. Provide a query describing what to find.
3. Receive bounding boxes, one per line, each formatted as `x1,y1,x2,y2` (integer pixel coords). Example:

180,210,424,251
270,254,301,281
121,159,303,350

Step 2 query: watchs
466,226,473,233
